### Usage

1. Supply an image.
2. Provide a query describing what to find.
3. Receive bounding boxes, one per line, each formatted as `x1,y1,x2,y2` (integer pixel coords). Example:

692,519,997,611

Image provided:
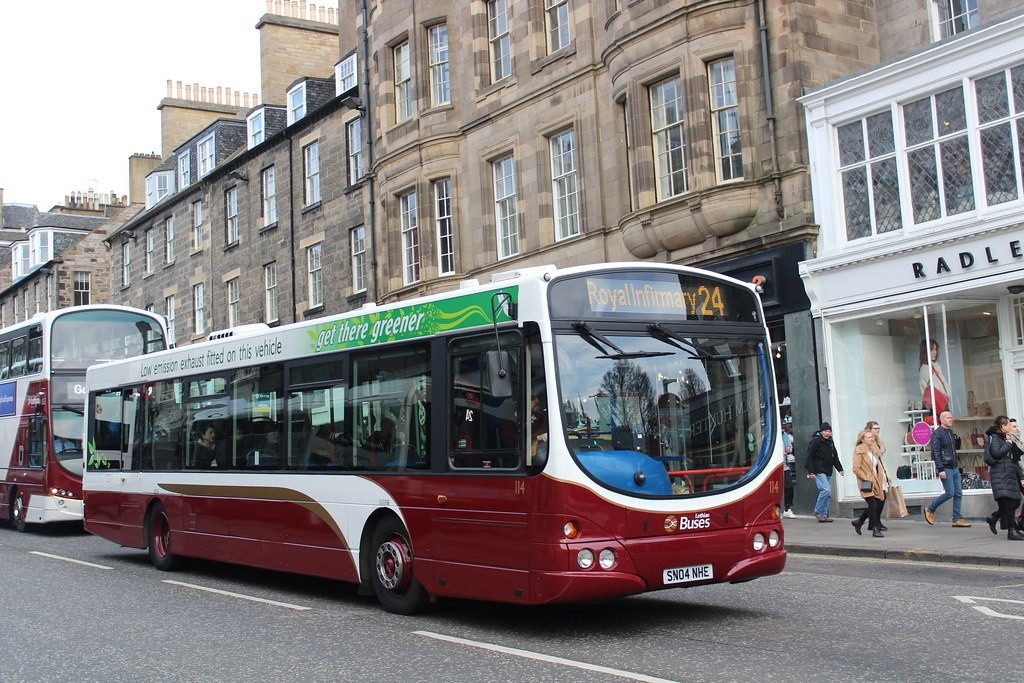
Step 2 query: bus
82,260,789,615
0,305,182,533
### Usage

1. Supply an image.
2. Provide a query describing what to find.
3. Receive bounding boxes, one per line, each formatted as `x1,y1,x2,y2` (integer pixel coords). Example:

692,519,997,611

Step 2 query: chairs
0,358,43,380
149,417,416,467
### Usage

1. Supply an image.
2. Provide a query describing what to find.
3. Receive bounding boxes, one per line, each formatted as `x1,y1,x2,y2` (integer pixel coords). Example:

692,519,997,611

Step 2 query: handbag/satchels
984,434,1006,466
897,365,993,489
861,480,872,492
886,481,909,518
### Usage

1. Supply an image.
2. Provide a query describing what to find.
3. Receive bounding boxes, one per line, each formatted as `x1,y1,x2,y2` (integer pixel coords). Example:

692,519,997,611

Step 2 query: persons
177,394,401,469
986,416,1024,540
851,421,892,537
918,339,951,414
924,412,972,527
500,387,547,466
781,425,796,518
808,422,844,521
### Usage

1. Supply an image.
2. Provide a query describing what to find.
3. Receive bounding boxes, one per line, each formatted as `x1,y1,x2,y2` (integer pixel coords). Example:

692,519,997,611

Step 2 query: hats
262,420,282,434
819,422,831,432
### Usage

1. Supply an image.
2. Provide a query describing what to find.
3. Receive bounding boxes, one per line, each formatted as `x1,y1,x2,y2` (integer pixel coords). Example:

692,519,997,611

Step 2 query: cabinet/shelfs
899,408,996,480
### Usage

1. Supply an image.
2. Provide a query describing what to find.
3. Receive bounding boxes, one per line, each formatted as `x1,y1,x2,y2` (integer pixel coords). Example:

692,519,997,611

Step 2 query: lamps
872,315,885,326
121,230,137,241
341,96,365,116
40,268,54,277
913,309,922,319
230,169,249,181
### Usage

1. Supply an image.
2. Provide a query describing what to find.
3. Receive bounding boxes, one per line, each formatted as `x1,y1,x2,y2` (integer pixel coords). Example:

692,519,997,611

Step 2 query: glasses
870,427,880,431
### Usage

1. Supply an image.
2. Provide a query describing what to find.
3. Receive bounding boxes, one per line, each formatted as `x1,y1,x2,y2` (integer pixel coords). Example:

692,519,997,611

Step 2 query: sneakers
951,520,971,526
925,507,933,525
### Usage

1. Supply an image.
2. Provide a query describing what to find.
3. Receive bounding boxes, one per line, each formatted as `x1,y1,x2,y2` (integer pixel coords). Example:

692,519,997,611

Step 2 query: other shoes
852,519,863,535
873,529,884,537
868,525,873,530
989,519,997,534
1007,526,1024,540
782,509,797,518
815,513,833,523
878,522,888,531
